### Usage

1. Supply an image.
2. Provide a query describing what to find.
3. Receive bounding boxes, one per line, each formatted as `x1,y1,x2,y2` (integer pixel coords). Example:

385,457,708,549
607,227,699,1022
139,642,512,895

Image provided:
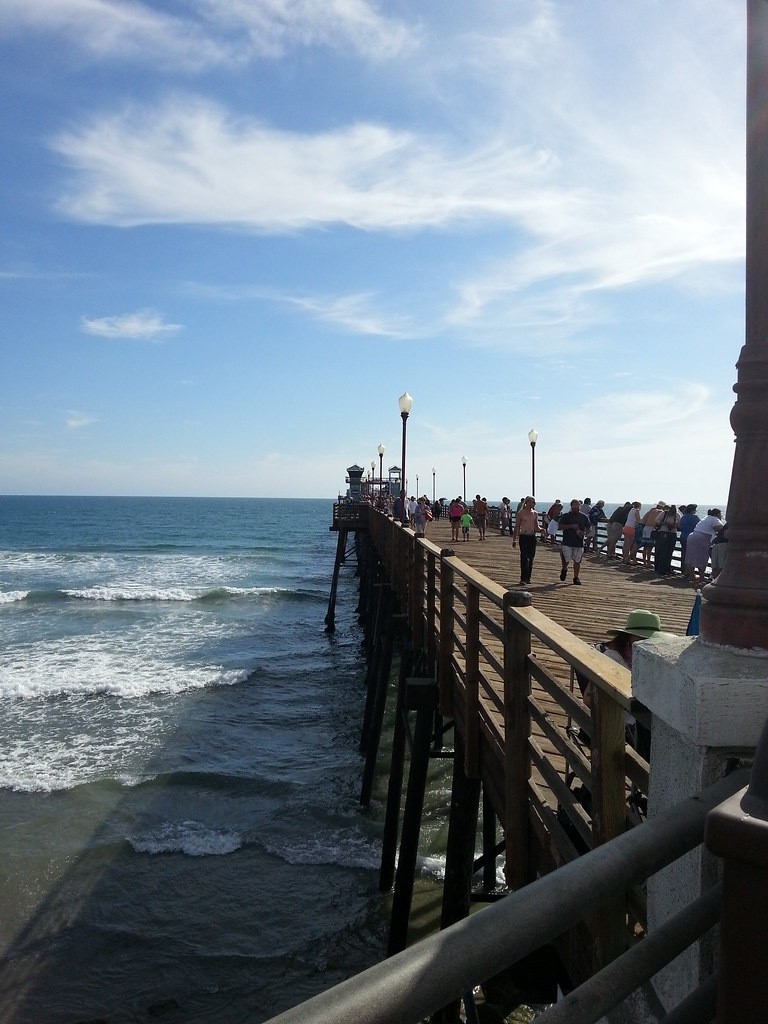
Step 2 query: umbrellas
439,497,447,501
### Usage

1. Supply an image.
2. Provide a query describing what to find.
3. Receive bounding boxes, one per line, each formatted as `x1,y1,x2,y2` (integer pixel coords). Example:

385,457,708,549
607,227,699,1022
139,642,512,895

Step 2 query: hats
607,609,679,639
410,496,416,500
654,501,666,508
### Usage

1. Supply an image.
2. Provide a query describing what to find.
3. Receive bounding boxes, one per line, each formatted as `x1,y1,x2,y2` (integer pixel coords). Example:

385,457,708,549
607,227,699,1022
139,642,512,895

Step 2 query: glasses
530,502,536,504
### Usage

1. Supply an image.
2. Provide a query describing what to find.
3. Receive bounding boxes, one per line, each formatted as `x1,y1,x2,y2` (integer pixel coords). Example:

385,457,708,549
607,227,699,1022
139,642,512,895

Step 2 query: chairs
564,663,651,826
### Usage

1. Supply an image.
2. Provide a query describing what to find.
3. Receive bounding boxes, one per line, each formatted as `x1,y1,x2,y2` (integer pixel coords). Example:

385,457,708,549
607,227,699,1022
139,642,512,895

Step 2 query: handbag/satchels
650,527,660,539
425,513,433,521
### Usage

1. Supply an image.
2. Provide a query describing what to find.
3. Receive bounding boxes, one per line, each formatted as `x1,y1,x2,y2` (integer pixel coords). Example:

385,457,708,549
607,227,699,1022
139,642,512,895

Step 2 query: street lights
431,467,437,506
377,444,385,511
366,470,372,501
460,455,469,502
405,479,409,497
398,391,413,528
370,461,376,508
415,474,420,499
528,428,539,498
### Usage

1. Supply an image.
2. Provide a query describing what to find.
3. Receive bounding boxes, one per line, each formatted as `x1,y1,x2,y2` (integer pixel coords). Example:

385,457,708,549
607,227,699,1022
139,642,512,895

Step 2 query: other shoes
540,538,547,543
462,540,465,542
585,547,654,568
467,539,470,541
451,538,455,540
479,538,482,541
656,566,710,583
573,577,581,585
526,580,532,584
520,581,525,585
483,538,487,540
501,531,505,536
550,541,557,545
560,570,567,581
456,540,460,542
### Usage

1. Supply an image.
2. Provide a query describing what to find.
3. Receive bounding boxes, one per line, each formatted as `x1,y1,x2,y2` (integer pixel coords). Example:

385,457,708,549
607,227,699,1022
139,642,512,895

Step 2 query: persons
581,608,661,750
558,498,591,585
539,499,563,545
579,498,728,582
448,494,489,542
498,496,525,537
360,492,445,527
413,496,430,534
513,495,546,586
